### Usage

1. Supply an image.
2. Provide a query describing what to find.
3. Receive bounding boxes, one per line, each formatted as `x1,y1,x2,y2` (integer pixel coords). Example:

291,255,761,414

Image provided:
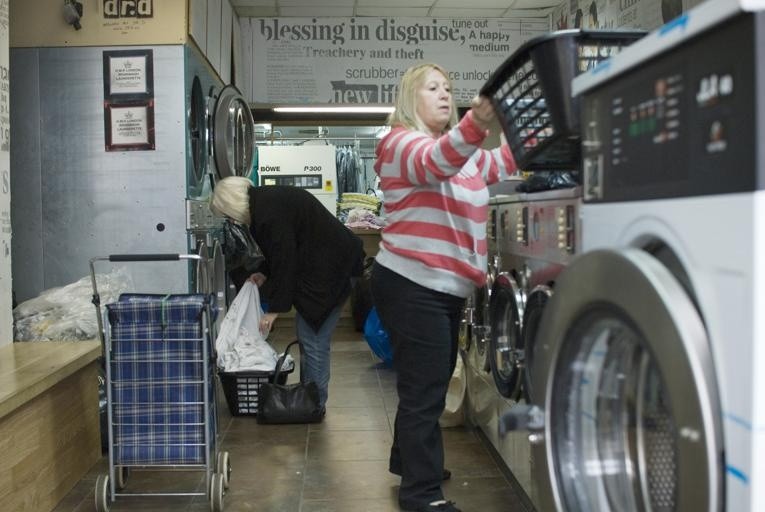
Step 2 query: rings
265,321,269,325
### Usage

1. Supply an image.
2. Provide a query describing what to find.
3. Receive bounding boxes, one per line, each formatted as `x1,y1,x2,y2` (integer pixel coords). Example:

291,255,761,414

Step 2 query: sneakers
415,498,463,511
388,463,451,483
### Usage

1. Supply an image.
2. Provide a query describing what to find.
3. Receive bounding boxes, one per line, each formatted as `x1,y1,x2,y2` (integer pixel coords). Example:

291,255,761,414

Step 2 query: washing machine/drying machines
463,188,582,512
529,0,765,512
9,48,256,339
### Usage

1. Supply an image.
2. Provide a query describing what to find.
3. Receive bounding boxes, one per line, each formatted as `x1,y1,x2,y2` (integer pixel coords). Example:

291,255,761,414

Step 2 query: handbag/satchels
257,340,324,425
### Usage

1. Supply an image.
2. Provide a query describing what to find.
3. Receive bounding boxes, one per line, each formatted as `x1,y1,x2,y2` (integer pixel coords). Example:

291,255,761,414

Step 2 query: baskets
217,361,295,418
478,27,654,172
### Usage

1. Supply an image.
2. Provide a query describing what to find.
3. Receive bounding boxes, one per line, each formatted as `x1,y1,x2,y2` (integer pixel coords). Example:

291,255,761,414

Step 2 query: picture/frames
102,48,155,152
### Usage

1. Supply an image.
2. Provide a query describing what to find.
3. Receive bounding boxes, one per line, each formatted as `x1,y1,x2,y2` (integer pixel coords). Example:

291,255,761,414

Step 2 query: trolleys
89,253,232,512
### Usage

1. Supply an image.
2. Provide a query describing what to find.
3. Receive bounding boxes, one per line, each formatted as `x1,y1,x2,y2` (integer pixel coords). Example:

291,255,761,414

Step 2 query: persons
213,177,367,416
370,63,553,512
